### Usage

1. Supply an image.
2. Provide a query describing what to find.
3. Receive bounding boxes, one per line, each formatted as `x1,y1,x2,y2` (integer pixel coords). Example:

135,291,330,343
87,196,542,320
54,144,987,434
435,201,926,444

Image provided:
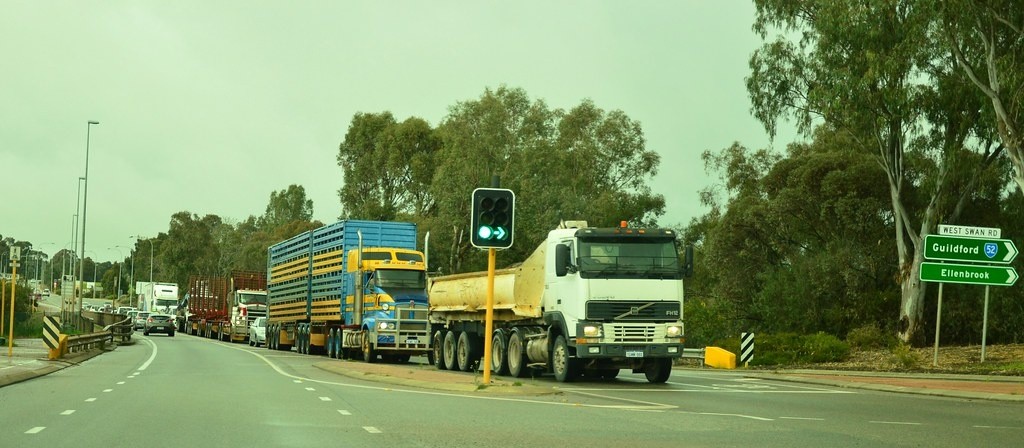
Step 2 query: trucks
136,281,179,328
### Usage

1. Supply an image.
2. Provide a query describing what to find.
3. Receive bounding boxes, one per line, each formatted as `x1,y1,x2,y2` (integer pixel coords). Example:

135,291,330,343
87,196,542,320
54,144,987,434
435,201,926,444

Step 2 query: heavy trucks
265,219,431,363
187,270,267,343
427,220,695,384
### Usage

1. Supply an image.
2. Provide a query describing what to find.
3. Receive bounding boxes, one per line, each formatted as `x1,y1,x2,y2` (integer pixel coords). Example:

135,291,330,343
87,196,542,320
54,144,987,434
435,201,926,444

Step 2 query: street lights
116,245,134,307
77,120,101,319
25,177,85,324
130,235,153,281
107,247,122,301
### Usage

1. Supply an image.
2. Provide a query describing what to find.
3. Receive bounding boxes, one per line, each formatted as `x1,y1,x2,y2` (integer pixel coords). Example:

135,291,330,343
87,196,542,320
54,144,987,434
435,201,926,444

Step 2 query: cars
81,302,136,324
133,311,152,331
42,289,50,297
249,317,265,347
143,314,175,337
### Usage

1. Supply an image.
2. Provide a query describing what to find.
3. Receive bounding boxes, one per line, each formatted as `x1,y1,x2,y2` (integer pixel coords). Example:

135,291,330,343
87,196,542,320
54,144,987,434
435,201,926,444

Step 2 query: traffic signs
919,262,1019,287
923,234,1019,266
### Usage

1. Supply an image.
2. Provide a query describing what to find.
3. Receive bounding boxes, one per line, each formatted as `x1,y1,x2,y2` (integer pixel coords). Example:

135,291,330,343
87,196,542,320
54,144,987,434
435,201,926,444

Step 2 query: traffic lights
469,188,514,251
